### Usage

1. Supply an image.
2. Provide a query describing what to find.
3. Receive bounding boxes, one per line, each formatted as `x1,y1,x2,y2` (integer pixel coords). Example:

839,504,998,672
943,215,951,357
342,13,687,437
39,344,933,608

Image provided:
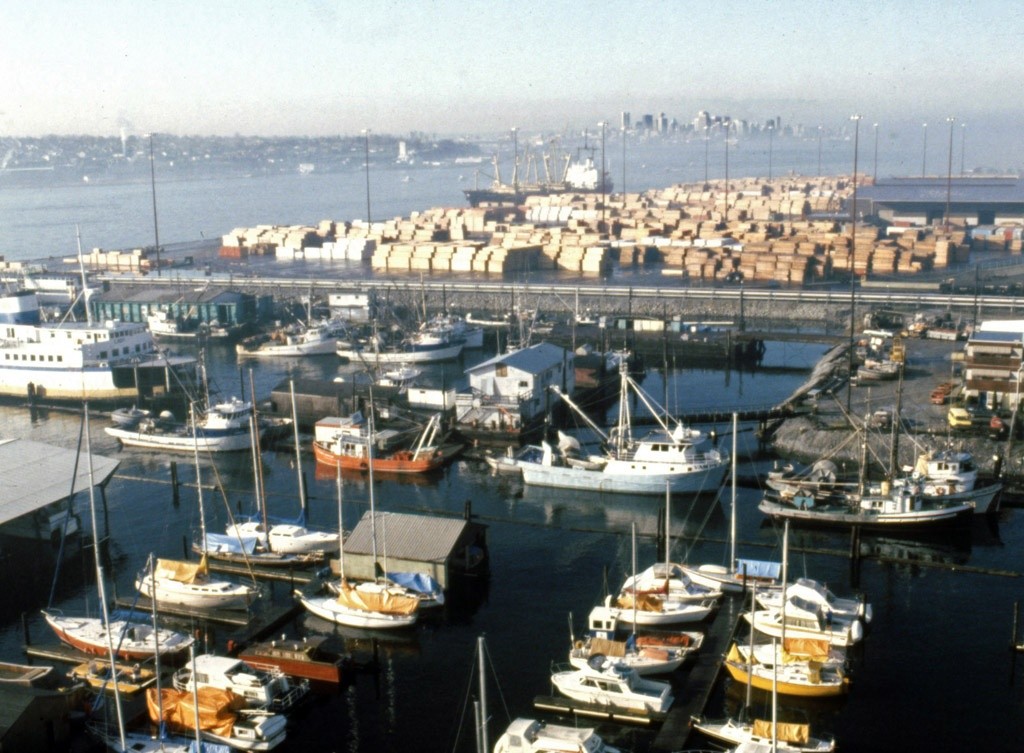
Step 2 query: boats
1,222,731,495
580,594,705,656
756,445,1004,532
742,594,865,648
723,641,850,698
752,578,874,624
621,562,724,607
461,149,615,208
493,716,621,753
549,658,676,714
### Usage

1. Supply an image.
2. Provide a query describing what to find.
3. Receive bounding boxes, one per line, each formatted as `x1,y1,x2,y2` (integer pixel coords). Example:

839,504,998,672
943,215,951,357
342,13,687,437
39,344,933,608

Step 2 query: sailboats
737,518,848,675
604,479,713,627
569,521,687,676
41,366,343,753
293,460,418,629
322,416,448,611
691,579,836,753
671,408,793,594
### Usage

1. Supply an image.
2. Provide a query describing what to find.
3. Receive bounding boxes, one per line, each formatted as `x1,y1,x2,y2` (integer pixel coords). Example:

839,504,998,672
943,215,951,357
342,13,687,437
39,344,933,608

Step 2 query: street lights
359,127,371,234
597,116,968,236
849,113,863,416
142,131,163,279
